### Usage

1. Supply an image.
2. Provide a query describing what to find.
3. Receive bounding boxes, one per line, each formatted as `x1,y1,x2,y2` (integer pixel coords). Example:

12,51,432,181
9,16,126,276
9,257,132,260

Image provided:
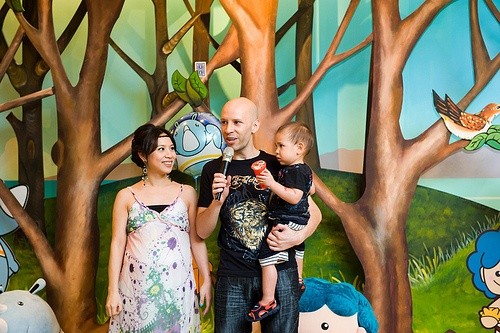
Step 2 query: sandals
296,279,307,298
243,298,282,322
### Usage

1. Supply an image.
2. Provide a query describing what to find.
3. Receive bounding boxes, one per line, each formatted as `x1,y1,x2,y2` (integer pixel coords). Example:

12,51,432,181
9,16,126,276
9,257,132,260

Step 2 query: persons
105,123,212,332
240,122,315,322
196,97,323,333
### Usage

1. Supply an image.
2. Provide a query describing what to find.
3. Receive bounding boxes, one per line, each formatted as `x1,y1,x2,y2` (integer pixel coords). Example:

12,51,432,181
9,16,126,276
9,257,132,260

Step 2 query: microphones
216,147,234,201
